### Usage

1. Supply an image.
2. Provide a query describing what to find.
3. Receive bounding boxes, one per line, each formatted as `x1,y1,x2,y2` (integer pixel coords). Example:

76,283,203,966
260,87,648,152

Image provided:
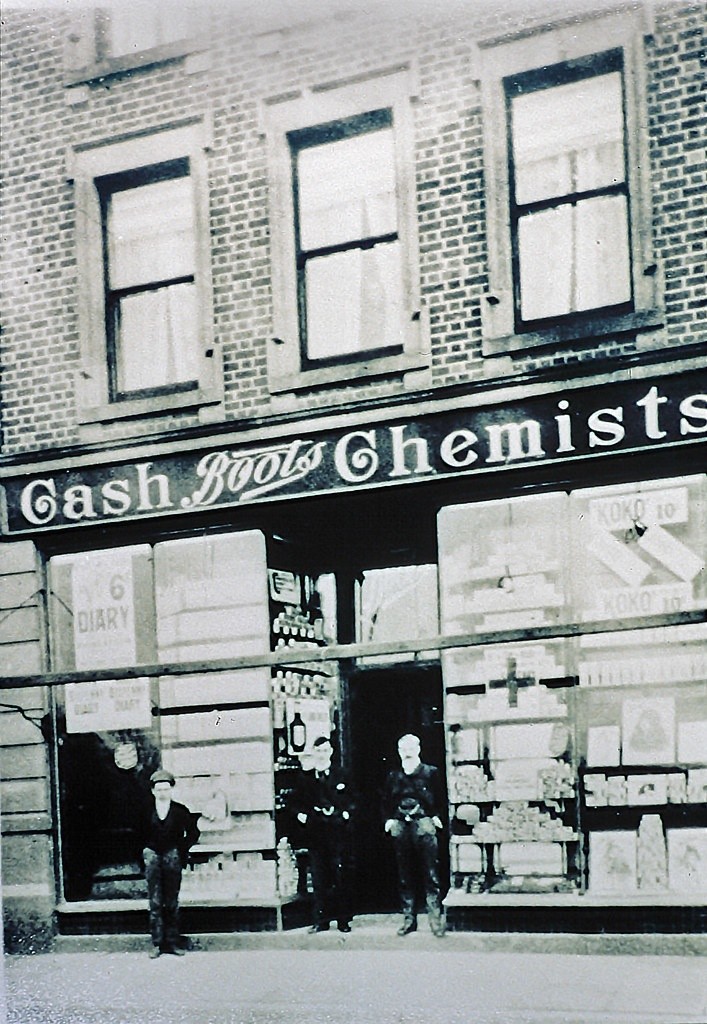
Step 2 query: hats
149,770,176,786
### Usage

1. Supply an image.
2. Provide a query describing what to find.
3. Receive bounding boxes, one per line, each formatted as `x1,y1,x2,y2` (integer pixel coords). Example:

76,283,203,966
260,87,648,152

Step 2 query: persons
130,769,202,958
384,732,452,937
296,734,359,934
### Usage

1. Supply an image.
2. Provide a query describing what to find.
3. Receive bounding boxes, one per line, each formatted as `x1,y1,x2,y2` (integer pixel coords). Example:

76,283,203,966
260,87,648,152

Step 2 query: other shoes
337,922,352,933
398,924,446,937
309,921,329,934
151,943,185,959
